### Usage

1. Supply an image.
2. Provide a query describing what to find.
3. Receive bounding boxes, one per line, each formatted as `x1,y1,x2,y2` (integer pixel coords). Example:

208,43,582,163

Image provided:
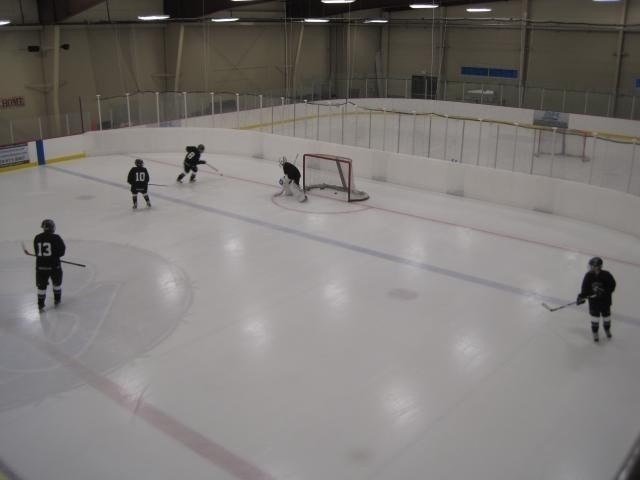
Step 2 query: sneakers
38,300,59,310
593,330,611,342
133,202,150,208
176,178,195,182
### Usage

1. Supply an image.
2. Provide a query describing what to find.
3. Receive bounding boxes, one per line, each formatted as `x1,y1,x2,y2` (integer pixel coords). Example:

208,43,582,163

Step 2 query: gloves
577,295,586,305
592,287,603,298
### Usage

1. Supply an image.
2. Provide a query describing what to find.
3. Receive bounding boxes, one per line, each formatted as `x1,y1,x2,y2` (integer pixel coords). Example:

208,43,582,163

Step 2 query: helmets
198,144,204,153
280,158,287,165
589,257,602,270
135,159,144,166
42,220,56,233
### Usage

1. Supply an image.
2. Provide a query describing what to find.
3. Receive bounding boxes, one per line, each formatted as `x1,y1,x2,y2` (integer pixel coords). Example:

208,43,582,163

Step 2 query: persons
575,256,616,344
34,219,67,310
278,156,306,202
127,158,153,210
176,143,207,184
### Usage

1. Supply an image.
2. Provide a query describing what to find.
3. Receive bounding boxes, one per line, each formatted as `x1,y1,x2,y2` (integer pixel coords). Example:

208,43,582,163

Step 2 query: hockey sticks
542,297,589,312
275,153,298,196
205,161,224,178
21,242,86,267
